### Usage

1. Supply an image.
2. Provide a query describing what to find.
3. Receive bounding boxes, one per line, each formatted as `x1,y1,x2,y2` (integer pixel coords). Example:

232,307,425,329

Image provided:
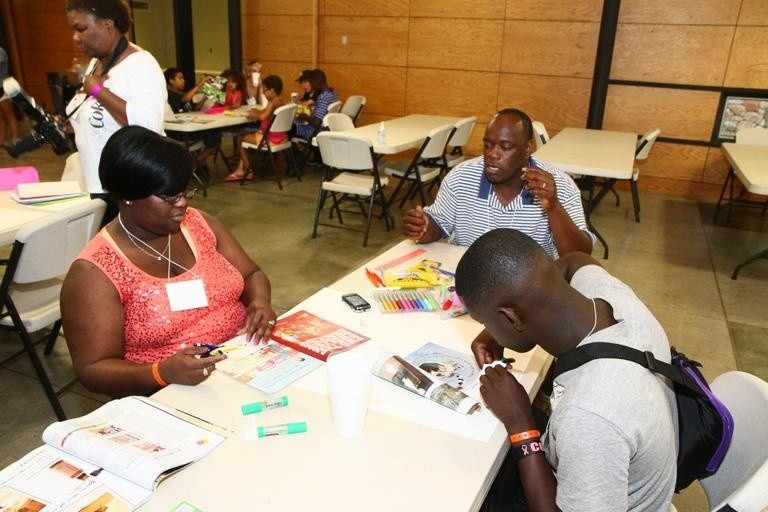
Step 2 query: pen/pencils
443,293,457,310
365,267,386,287
200,350,224,359
375,289,438,311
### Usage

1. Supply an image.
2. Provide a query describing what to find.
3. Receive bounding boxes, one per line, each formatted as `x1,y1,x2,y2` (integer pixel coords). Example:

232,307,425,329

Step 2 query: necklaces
573,293,600,349
121,226,176,262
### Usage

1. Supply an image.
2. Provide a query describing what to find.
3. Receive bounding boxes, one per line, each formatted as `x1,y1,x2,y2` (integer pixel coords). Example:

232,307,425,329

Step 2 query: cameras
3,76,72,159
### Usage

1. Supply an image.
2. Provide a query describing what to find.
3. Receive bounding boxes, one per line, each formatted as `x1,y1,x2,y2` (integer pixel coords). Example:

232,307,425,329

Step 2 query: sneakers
285,155,326,178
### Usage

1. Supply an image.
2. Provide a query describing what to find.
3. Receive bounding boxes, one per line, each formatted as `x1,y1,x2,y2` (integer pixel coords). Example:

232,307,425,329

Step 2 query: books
0,395,230,512
370,338,498,437
9,179,88,207
207,308,372,398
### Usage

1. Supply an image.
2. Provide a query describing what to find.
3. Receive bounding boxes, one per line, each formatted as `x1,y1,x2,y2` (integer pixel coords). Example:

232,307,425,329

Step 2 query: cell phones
342,294,371,310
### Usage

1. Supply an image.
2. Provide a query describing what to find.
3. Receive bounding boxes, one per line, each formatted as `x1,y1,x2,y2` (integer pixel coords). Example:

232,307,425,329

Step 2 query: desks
0,238,554,512
532,126,637,259
350,114,467,155
714,143,767,280
165,108,260,190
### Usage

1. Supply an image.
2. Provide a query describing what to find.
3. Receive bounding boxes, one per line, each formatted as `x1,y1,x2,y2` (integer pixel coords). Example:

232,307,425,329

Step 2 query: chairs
380,124,452,209
736,127,766,143
3,153,93,243
531,119,549,147
681,371,766,511
607,129,661,223
296,93,367,133
239,103,302,188
429,116,478,193
311,132,391,246
3,199,106,420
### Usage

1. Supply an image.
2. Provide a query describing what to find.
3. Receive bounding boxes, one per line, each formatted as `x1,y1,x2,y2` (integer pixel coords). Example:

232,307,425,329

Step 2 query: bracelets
89,82,106,99
508,442,545,461
508,428,542,443
151,358,169,389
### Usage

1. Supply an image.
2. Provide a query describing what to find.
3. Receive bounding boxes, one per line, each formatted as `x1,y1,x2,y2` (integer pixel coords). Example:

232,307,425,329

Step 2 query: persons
57,123,277,404
0,47,9,99
59,1,170,241
164,59,340,185
400,107,597,263
454,227,680,509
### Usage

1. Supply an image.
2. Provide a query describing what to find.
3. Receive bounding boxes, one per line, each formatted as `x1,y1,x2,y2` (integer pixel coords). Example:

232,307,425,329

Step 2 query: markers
242,395,288,416
257,422,307,438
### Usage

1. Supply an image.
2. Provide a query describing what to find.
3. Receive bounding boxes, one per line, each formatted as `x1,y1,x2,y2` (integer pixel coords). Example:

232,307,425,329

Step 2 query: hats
294,70,313,81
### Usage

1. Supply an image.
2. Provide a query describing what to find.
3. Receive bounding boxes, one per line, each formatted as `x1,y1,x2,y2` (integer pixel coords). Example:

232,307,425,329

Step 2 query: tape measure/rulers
376,248,427,271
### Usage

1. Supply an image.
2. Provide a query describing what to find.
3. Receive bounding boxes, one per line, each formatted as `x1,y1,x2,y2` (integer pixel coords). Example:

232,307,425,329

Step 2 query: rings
542,183,546,190
267,319,275,327
201,366,209,377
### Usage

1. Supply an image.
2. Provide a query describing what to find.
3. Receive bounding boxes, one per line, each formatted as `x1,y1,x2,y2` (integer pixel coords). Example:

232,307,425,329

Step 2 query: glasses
153,183,198,204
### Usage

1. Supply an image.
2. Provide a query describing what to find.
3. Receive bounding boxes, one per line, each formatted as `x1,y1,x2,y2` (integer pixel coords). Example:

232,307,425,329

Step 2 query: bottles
377,119,385,143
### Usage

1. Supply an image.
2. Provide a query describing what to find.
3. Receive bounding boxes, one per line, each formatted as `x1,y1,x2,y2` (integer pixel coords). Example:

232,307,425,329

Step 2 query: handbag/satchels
664,344,735,494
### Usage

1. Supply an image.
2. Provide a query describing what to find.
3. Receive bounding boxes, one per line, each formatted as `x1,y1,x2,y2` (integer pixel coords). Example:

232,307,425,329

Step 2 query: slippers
246,168,256,180
224,173,245,181
